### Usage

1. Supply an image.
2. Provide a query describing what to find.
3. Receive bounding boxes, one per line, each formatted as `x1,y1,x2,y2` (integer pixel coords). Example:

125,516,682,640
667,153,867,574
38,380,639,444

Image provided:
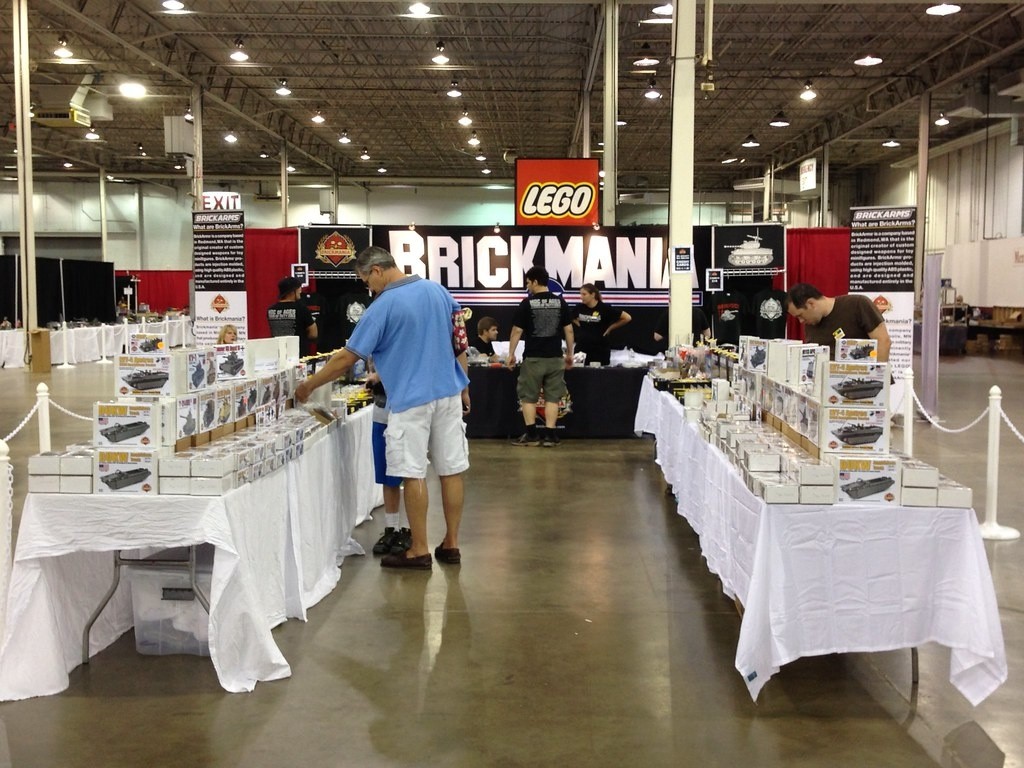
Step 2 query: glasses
364,269,372,290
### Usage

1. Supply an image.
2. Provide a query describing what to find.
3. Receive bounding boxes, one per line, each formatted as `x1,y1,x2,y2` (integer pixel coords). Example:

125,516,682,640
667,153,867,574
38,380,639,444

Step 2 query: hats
278,277,306,297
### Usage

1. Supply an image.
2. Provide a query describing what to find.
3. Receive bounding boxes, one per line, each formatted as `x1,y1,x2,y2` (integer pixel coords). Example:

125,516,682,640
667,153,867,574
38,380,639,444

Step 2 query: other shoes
434,538,461,564
381,550,433,569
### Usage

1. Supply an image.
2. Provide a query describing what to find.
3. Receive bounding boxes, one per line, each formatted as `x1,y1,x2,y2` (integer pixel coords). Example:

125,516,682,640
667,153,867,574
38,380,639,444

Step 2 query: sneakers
542,435,564,446
372,527,402,554
510,432,541,446
391,526,413,554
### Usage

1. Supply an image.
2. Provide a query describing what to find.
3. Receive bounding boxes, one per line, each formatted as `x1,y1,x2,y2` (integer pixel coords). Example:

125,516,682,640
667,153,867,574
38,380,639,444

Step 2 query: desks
913,325,968,356
634,376,1008,709
0,403,383,703
462,363,653,442
0,317,192,369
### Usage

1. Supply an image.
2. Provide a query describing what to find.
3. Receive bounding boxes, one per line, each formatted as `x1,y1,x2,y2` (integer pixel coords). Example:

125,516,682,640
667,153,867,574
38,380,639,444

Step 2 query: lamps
53,0,964,185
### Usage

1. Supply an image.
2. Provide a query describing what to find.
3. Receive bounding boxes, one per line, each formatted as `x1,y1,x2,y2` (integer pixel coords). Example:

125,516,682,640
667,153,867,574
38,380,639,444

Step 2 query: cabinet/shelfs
941,287,968,326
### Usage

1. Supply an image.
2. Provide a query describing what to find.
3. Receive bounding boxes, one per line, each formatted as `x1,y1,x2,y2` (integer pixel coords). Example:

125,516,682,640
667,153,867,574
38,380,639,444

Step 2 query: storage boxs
651,334,972,510
27,335,370,497
127,542,218,657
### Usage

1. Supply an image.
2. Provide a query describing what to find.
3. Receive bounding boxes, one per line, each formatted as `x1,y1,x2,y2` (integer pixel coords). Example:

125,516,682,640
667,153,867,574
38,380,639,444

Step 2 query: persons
946,296,973,324
654,307,711,352
785,283,895,384
294,248,471,568
266,276,318,359
218,325,237,345
570,283,631,365
506,265,574,447
466,317,499,363
